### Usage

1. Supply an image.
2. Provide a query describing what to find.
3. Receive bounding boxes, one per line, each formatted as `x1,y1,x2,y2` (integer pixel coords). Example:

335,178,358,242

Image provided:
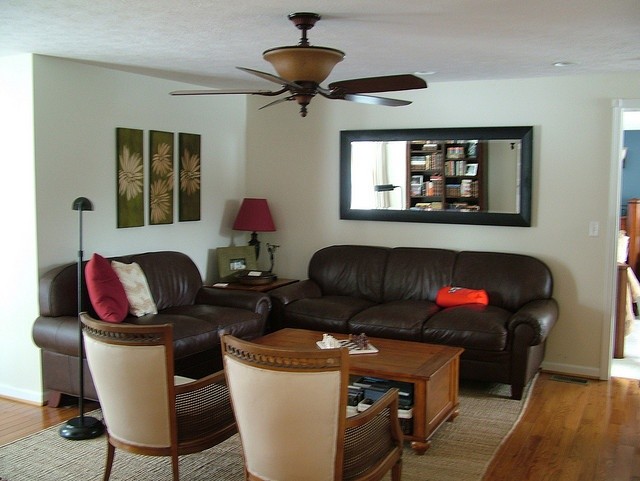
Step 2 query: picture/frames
217,245,258,279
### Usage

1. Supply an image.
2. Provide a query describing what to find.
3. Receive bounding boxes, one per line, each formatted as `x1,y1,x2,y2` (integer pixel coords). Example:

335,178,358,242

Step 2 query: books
446,180,478,198
423,144,437,151
411,153,443,170
445,161,466,177
446,147,465,158
411,175,443,197
410,201,443,211
447,202,480,212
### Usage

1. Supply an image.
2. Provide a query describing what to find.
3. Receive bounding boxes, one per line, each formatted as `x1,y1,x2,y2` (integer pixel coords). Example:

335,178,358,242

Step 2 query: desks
203,275,300,292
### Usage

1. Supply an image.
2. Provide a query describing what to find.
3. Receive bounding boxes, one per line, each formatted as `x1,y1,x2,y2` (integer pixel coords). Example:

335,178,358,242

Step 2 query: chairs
221,340,408,480
77,311,239,481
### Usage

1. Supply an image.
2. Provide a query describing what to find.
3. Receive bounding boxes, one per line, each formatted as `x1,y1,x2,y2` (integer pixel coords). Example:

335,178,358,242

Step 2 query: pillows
84,251,129,322
435,285,489,306
110,259,158,316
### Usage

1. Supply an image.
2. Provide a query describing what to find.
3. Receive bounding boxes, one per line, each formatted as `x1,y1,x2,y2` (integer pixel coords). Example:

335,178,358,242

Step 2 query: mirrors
339,125,533,227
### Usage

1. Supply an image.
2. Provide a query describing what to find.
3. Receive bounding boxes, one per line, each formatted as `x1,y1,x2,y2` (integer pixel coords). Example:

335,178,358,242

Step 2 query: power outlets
588,222,600,238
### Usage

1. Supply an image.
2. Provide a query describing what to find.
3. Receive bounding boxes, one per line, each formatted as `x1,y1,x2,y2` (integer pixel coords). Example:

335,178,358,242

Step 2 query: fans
168,12,427,117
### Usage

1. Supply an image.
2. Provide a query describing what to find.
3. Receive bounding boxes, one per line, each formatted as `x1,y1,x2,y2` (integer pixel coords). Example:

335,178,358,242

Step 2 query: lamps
232,199,276,260
58,195,104,440
372,183,404,208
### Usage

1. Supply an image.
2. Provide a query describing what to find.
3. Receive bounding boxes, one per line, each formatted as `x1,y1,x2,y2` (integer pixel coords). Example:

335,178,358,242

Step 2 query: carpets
0,368,541,480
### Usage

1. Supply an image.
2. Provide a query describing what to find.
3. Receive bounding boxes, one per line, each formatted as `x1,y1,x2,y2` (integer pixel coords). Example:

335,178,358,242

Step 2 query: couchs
32,246,273,411
269,245,560,399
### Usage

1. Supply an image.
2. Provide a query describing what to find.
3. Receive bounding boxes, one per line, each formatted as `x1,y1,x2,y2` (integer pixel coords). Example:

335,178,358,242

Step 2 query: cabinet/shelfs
406,141,489,212
